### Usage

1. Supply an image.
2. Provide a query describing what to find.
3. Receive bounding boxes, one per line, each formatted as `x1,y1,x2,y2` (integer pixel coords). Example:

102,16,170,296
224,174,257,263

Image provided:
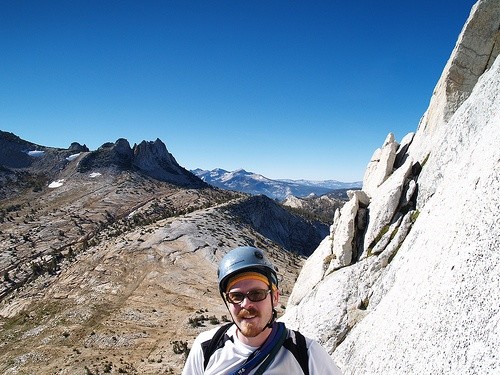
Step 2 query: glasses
225,288,270,304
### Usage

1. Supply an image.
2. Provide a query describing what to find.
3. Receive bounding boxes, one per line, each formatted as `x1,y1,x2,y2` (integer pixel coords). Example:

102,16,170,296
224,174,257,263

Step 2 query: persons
180,247,343,375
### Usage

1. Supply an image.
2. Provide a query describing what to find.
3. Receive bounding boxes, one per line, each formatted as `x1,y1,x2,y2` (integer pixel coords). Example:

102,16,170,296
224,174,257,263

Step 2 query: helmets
216,246,279,292
